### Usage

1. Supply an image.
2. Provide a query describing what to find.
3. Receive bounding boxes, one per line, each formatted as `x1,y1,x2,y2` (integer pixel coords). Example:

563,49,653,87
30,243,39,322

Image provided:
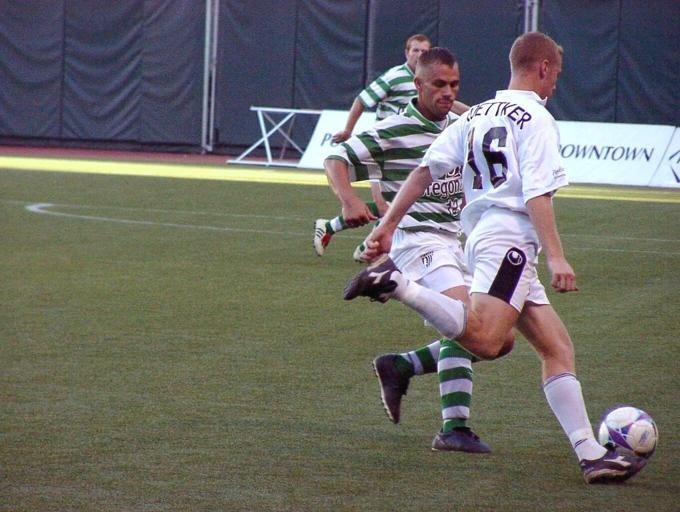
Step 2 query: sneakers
312,219,330,257
579,450,646,484
344,253,402,303
431,426,492,453
373,354,409,424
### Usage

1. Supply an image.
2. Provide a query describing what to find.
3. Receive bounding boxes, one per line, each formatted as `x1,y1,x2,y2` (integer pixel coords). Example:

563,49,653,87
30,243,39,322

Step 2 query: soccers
598,407,659,459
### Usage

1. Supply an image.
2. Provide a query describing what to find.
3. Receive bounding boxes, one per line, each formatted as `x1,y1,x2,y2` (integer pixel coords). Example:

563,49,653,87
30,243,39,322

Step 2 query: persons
343,31,646,484
313,34,471,261
323,47,515,453
